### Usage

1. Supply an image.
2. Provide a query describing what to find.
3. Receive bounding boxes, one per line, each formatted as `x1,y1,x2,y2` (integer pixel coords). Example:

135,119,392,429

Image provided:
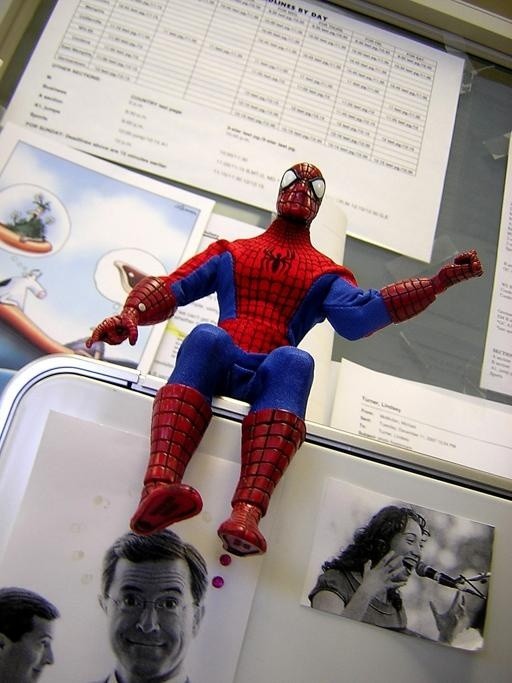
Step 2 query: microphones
415,561,468,592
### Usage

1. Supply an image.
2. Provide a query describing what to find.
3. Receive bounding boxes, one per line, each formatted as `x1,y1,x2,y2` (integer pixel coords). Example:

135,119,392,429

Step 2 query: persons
305,504,469,646
0,585,62,683
93,529,206,682
85,160,484,560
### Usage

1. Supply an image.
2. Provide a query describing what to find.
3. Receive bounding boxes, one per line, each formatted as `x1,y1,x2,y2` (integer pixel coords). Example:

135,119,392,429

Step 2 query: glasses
104,592,195,615
